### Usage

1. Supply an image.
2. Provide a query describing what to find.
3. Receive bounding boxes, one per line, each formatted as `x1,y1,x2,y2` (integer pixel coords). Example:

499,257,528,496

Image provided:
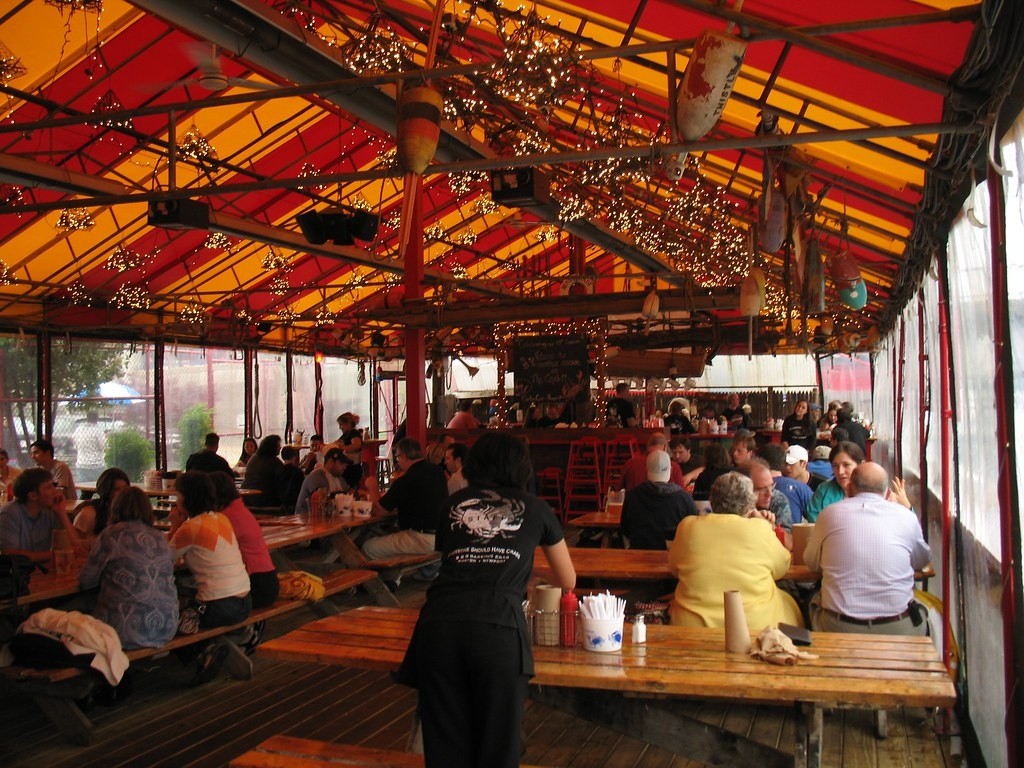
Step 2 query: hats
325,448,353,465
785,445,808,464
810,404,822,411
814,446,832,459
647,450,671,483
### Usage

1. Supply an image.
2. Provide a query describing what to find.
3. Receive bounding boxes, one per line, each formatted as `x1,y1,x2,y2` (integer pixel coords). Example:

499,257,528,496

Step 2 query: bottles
775,522,785,547
631,613,646,643
559,589,576,647
7,482,13,502
310,491,320,515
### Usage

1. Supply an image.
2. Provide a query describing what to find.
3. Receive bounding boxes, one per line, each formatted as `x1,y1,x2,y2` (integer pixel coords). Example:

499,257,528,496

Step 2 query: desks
258,489,441,608
534,546,936,624
567,509,623,528
253,604,960,768
0,524,345,747
75,481,261,501
64,499,87,513
281,439,389,472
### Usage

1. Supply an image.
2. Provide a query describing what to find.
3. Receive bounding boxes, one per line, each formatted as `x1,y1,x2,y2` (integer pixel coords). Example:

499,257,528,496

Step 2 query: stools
536,435,641,525
372,455,390,491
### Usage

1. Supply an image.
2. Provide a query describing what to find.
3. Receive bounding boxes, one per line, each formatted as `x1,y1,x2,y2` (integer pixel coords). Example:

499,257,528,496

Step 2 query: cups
56,550,72,578
605,492,624,518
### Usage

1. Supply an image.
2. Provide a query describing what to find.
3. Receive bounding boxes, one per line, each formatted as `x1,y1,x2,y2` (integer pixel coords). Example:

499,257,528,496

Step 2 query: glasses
754,481,777,495
393,451,408,462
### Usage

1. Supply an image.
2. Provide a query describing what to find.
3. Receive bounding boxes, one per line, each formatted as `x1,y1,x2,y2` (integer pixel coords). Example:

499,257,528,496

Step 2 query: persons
0,448,23,492
30,438,77,499
446,399,481,446
73,468,130,535
394,434,577,768
186,433,240,478
805,441,865,523
0,467,81,682
619,393,872,500
735,460,793,551
620,449,699,550
167,470,252,687
605,383,638,427
667,470,805,630
753,443,814,523
802,462,932,635
235,412,470,593
539,403,573,427
71,487,179,710
210,473,280,654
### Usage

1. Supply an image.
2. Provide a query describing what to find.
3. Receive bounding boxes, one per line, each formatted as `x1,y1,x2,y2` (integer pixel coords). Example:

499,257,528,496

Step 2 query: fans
131,43,282,92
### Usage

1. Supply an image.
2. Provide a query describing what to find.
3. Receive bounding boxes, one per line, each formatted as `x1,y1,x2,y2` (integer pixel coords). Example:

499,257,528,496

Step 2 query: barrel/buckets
695,501,712,516
292,433,301,446
582,614,625,652
337,497,352,515
353,501,373,517
710,425,728,434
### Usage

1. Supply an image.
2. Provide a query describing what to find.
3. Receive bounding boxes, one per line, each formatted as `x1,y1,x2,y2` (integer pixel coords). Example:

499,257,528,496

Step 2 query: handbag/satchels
0,553,36,605
277,570,325,600
178,608,199,633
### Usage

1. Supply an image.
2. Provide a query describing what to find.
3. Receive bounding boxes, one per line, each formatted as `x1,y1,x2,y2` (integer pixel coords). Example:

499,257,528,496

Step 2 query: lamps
295,102,380,245
314,340,324,362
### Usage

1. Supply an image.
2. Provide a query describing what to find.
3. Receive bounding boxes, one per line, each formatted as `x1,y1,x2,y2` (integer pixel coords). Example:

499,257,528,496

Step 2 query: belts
825,609,909,625
409,527,437,535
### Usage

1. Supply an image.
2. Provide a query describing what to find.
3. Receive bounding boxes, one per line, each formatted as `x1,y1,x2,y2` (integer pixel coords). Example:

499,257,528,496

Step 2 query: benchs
9,570,399,681
363,553,441,570
156,499,177,504
152,520,172,529
230,734,539,768
151,505,172,511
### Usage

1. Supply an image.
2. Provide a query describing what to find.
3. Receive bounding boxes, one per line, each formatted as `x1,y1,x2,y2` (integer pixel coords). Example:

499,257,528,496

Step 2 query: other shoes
384,580,397,592
69,619,265,711
413,571,439,581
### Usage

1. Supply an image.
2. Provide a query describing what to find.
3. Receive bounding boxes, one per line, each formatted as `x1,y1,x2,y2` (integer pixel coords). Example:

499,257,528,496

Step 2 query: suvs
74,414,181,454
12,417,77,467
